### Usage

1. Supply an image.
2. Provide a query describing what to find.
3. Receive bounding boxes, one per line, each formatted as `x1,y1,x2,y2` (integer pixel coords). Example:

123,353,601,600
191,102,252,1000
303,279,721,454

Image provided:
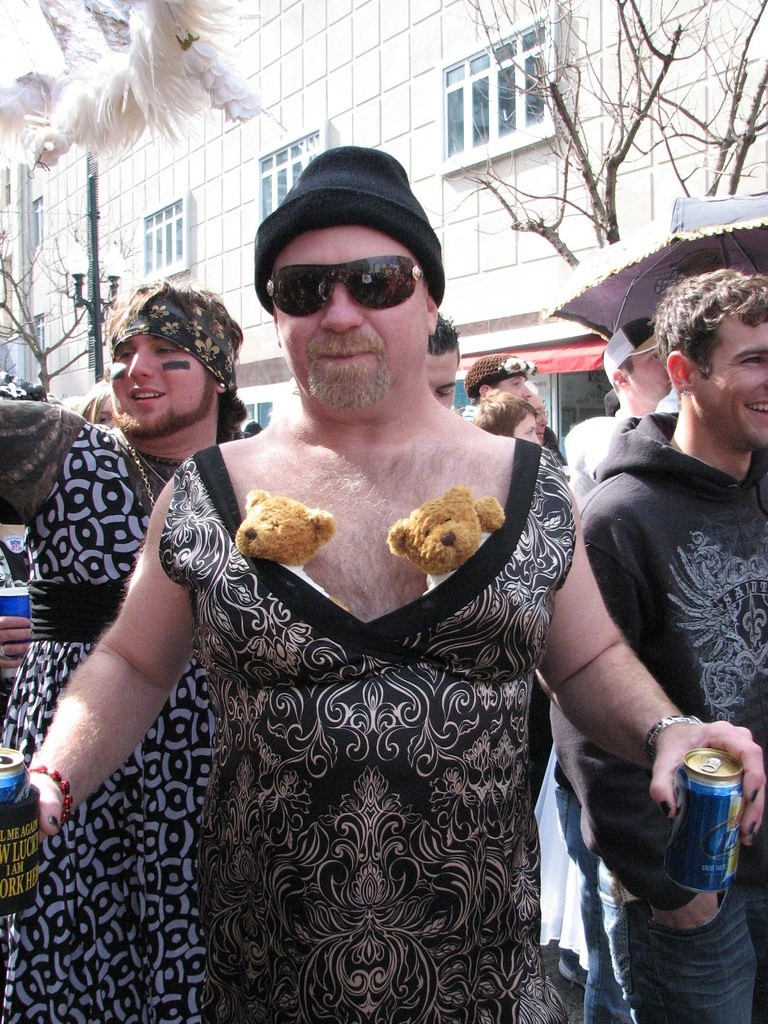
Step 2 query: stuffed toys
235,489,350,616
387,487,505,596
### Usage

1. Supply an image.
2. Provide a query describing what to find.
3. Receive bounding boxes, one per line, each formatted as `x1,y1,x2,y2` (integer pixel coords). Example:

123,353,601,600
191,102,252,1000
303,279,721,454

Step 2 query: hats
464,355,538,398
603,316,662,385
254,146,445,323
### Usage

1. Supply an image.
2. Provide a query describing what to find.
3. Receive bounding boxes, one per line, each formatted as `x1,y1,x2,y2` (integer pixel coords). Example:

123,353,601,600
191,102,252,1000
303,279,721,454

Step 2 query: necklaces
128,445,170,509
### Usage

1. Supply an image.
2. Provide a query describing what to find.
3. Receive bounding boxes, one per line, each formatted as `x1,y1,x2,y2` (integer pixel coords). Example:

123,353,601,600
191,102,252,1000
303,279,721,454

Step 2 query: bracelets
641,715,704,770
28,761,74,832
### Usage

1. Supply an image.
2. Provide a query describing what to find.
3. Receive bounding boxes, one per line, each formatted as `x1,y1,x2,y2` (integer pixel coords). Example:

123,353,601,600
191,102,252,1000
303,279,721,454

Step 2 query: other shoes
559,955,587,990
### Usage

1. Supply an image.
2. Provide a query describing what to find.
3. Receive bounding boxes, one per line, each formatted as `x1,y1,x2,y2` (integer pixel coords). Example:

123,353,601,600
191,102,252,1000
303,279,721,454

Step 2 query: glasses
535,409,548,426
265,255,424,316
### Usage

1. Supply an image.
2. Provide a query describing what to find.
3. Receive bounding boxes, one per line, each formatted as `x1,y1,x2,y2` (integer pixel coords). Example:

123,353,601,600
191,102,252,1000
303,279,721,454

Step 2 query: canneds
664,748,744,894
0,748,31,804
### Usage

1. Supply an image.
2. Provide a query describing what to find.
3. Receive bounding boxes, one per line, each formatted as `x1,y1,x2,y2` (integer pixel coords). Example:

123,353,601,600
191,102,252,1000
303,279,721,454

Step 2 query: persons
0,148,768,1024
551,269,768,1024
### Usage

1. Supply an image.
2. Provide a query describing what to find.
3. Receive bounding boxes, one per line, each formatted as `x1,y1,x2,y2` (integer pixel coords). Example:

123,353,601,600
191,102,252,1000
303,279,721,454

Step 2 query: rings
0,646,10,658
